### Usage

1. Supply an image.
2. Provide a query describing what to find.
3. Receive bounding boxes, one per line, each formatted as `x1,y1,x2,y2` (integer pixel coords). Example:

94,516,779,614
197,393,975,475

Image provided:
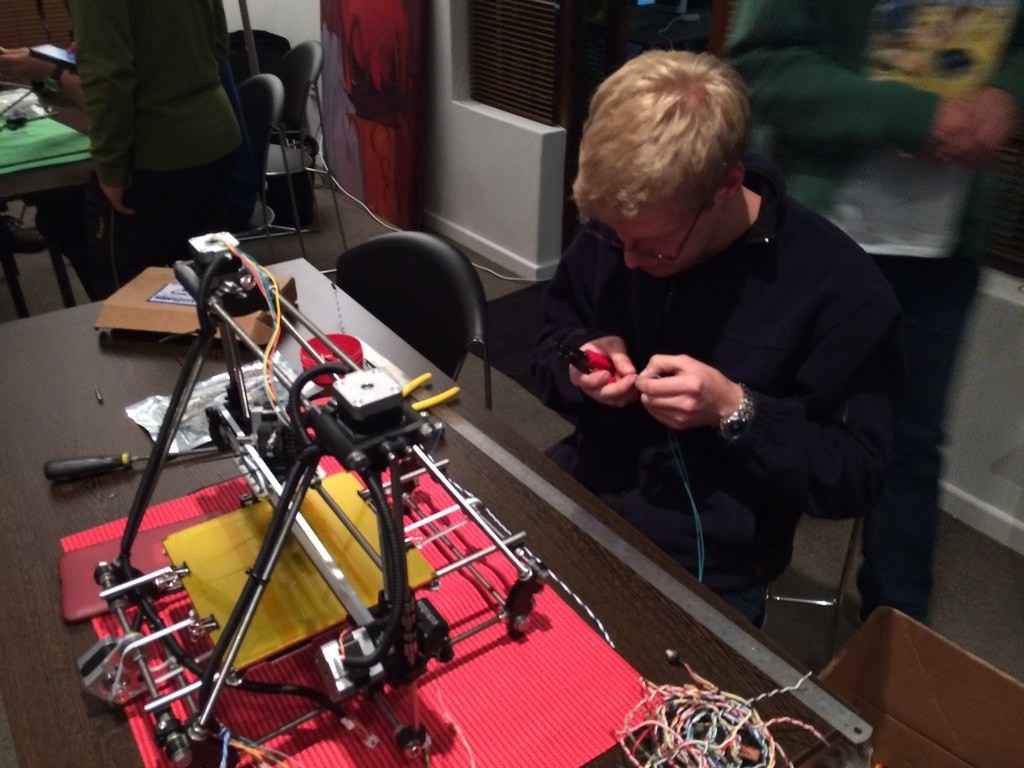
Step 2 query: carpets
468,281,576,427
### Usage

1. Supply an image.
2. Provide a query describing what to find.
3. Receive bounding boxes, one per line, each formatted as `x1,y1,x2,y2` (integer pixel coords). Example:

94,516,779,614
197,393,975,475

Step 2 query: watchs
43,64,65,95
720,383,754,441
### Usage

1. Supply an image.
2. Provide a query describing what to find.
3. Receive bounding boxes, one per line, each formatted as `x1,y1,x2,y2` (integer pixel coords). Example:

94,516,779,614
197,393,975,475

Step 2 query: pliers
402,372,460,411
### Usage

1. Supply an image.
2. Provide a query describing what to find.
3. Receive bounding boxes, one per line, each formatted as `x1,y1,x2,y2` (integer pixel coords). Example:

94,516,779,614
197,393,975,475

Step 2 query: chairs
752,505,868,665
233,40,350,264
333,232,491,407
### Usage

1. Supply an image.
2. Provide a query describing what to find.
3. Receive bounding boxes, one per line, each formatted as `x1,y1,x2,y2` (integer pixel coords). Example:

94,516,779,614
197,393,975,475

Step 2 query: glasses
581,199,708,265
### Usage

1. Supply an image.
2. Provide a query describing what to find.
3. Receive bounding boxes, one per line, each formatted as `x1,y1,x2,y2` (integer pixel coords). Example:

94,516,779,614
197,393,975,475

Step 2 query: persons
719,0,1024,631
70,0,245,303
526,48,900,636
0,47,115,303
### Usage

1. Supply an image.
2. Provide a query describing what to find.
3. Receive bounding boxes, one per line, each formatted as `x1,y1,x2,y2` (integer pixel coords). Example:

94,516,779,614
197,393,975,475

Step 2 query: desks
0,258,879,768
0,77,100,320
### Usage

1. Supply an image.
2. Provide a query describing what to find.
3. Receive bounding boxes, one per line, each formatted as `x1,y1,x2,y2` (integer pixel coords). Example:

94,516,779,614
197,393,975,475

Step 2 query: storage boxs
93,266,299,347
814,603,1024,768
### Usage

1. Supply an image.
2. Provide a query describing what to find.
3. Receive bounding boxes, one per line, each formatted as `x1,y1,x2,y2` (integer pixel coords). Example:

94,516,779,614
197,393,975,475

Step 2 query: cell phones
30,44,76,72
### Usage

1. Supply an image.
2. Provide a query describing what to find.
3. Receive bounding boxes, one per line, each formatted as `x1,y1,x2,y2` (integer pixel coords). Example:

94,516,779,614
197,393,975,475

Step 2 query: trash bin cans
264,130,319,228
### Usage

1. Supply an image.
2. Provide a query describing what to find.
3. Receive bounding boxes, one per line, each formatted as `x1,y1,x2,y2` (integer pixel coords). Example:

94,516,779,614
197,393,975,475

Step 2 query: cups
300,334,364,384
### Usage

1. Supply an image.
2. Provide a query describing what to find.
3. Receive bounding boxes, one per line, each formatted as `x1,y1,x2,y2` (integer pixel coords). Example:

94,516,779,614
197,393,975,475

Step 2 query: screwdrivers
42,445,220,481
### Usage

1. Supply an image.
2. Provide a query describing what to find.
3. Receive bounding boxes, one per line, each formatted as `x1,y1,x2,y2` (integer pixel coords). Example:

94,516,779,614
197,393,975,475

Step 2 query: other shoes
2,214,45,252
857,605,933,629
